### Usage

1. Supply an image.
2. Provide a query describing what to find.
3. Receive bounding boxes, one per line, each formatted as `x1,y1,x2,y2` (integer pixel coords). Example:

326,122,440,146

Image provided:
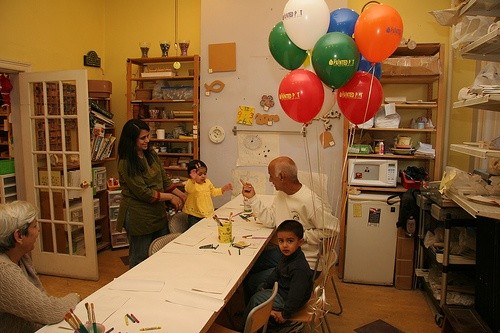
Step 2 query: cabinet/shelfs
338,42,445,278
127,55,201,186
88,80,118,164
0,172,17,204
0,106,15,160
107,189,130,248
37,161,111,256
443,0,500,221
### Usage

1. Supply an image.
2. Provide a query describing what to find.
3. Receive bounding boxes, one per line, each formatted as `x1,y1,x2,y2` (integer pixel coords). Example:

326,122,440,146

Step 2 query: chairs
207,282,278,333
168,212,189,233
270,232,343,333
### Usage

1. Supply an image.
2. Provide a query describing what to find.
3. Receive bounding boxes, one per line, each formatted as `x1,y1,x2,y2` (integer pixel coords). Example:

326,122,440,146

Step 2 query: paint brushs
63,302,103,333
212,213,233,227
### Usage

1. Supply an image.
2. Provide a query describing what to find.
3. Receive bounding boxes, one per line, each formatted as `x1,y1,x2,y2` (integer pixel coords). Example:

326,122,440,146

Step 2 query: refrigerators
344,193,401,286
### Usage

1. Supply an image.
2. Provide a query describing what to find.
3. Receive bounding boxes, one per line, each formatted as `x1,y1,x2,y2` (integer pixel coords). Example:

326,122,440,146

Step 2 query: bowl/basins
135,88,152,101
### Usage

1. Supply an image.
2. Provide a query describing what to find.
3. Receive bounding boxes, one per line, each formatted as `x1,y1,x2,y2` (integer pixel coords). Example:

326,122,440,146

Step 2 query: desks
35,193,277,333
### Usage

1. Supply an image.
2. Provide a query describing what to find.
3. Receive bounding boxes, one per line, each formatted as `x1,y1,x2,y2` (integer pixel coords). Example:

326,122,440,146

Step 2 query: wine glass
139,40,151,58
160,40,171,57
178,39,190,56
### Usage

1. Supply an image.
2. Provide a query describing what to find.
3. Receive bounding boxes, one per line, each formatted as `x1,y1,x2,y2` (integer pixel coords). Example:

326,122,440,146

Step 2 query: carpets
354,319,403,333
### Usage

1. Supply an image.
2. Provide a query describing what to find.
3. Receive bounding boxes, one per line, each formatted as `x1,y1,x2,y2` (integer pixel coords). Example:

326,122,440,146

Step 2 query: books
61,103,116,201
479,84,500,95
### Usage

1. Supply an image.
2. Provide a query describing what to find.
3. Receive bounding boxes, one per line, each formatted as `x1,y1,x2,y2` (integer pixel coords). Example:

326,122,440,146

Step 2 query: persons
239,156,340,294
241,220,312,333
182,159,232,228
115,119,187,269
0,200,80,333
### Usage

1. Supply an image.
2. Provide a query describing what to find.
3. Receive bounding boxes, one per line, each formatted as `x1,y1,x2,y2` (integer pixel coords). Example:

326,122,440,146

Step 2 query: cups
218,221,232,243
160,147,166,152
74,322,105,333
156,128,165,139
154,149,159,153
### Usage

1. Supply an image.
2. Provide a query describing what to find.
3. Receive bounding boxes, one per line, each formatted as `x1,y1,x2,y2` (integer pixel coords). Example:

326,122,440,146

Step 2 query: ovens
346,157,397,188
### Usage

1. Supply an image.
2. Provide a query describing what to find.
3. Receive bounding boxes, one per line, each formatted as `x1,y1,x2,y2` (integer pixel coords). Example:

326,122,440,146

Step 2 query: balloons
278,69,324,124
314,82,336,120
268,22,307,71
312,32,359,91
358,54,381,80
283,0,330,50
354,3,403,66
327,8,360,37
337,70,382,128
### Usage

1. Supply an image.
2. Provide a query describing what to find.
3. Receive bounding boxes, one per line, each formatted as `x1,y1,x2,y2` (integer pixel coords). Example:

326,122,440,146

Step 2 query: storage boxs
141,72,177,77
347,144,370,153
382,52,441,76
94,166,107,191
160,86,194,100
395,227,414,291
63,198,100,231
400,170,428,189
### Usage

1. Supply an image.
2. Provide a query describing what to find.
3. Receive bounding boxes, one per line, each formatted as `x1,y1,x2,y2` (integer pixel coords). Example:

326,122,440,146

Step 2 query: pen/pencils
227,236,241,256
214,245,220,250
242,235,253,238
239,212,257,223
123,311,139,326
140,326,161,332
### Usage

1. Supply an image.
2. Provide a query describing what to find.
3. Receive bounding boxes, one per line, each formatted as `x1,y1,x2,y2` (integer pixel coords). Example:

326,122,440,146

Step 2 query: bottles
149,105,158,119
